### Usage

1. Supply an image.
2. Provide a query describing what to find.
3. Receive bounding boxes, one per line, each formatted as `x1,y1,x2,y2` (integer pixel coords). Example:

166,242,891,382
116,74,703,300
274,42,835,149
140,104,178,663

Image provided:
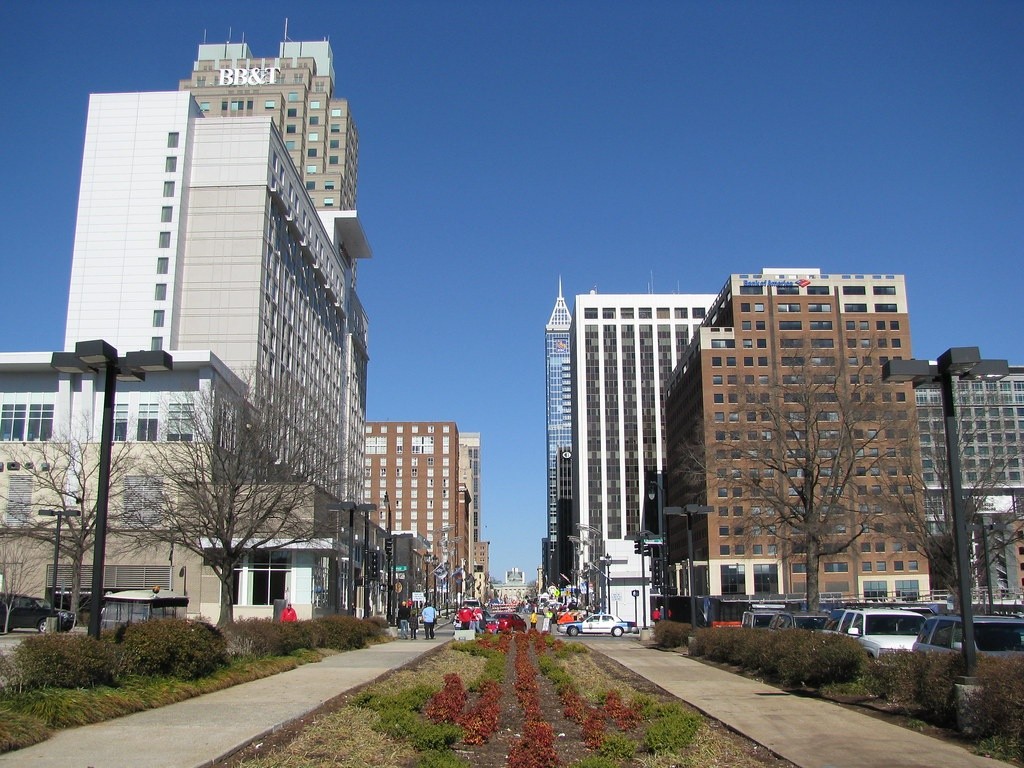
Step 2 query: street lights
605,554,612,614
444,560,450,618
880,345,1011,684
383,491,394,623
423,551,432,605
327,501,377,620
48,339,173,642
663,503,715,629
454,563,459,611
584,564,590,609
38,509,80,617
648,481,670,589
432,554,439,609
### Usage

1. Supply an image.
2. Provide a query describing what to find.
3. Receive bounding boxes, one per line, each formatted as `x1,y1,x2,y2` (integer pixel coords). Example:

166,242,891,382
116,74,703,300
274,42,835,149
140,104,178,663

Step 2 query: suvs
843,605,936,617
914,616,1024,657
0,592,77,632
462,598,481,611
822,609,927,659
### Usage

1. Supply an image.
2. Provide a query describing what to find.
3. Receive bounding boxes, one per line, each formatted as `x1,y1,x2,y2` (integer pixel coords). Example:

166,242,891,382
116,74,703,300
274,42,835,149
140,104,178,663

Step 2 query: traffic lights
385,538,392,556
633,591,638,596
644,542,651,557
635,540,641,554
380,586,385,590
368,552,377,578
652,559,662,588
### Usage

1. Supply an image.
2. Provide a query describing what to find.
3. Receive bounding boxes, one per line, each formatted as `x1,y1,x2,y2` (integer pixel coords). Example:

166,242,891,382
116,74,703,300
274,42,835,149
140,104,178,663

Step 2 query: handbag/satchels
433,618,437,624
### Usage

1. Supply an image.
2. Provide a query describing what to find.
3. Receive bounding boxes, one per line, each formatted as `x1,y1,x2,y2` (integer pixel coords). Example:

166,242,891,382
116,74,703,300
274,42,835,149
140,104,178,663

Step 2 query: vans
741,611,778,631
538,597,548,613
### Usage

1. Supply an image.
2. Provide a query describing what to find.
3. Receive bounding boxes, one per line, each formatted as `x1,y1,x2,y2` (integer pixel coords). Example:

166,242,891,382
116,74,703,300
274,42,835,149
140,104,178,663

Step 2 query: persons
459,603,482,634
546,608,553,633
543,604,565,624
398,601,410,640
408,611,418,640
653,608,660,623
420,601,423,608
280,602,297,622
422,602,436,640
408,598,412,608
660,606,671,621
529,610,537,629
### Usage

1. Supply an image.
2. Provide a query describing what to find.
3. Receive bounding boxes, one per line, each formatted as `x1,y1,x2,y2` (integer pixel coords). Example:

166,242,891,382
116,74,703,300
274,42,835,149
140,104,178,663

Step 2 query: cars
768,610,831,632
556,615,637,637
485,614,527,634
